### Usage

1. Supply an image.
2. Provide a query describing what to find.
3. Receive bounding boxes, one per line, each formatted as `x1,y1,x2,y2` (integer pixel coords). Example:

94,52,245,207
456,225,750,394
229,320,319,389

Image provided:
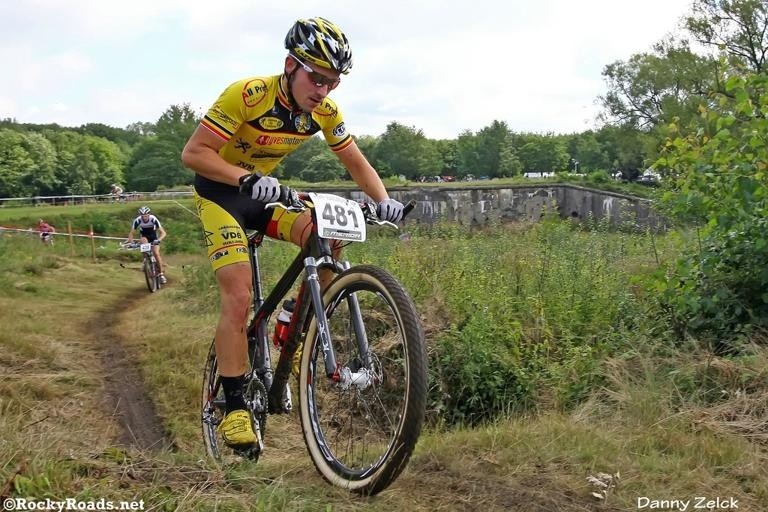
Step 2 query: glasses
287,53,340,90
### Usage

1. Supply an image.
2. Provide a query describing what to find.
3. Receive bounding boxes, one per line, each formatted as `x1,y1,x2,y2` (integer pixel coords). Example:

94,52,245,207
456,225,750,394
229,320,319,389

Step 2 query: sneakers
215,408,257,446
159,274,167,283
290,333,307,379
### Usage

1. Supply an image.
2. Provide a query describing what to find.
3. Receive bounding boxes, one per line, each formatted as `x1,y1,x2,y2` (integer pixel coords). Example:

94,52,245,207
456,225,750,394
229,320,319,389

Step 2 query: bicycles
121,241,165,293
193,198,429,498
107,193,129,204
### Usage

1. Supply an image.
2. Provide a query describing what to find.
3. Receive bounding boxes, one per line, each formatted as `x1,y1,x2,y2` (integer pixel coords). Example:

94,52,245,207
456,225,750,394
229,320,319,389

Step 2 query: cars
415,174,490,182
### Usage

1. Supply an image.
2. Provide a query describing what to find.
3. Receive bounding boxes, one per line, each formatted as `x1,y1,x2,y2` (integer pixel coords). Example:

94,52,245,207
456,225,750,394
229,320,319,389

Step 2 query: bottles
271,299,297,349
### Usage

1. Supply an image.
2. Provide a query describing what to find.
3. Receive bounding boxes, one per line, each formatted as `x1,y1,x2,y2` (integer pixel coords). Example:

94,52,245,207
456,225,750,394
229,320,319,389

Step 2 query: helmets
285,17,352,75
138,206,150,215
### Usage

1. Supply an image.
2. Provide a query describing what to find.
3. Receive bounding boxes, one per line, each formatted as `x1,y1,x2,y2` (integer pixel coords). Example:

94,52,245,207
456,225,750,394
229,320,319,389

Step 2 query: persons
181,18,405,452
33,219,55,245
129,206,167,285
110,184,123,200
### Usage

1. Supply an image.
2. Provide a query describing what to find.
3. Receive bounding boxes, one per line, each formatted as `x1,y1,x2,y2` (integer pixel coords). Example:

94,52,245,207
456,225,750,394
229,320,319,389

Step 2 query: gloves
244,170,282,203
377,198,406,224
